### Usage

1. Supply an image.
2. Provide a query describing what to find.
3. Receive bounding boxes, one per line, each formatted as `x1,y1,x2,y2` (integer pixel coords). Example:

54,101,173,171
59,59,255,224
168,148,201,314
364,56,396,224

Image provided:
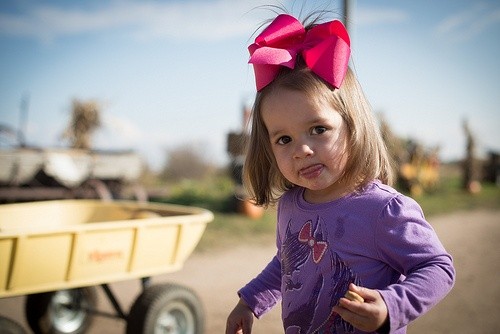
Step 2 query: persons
226,0,456,334
